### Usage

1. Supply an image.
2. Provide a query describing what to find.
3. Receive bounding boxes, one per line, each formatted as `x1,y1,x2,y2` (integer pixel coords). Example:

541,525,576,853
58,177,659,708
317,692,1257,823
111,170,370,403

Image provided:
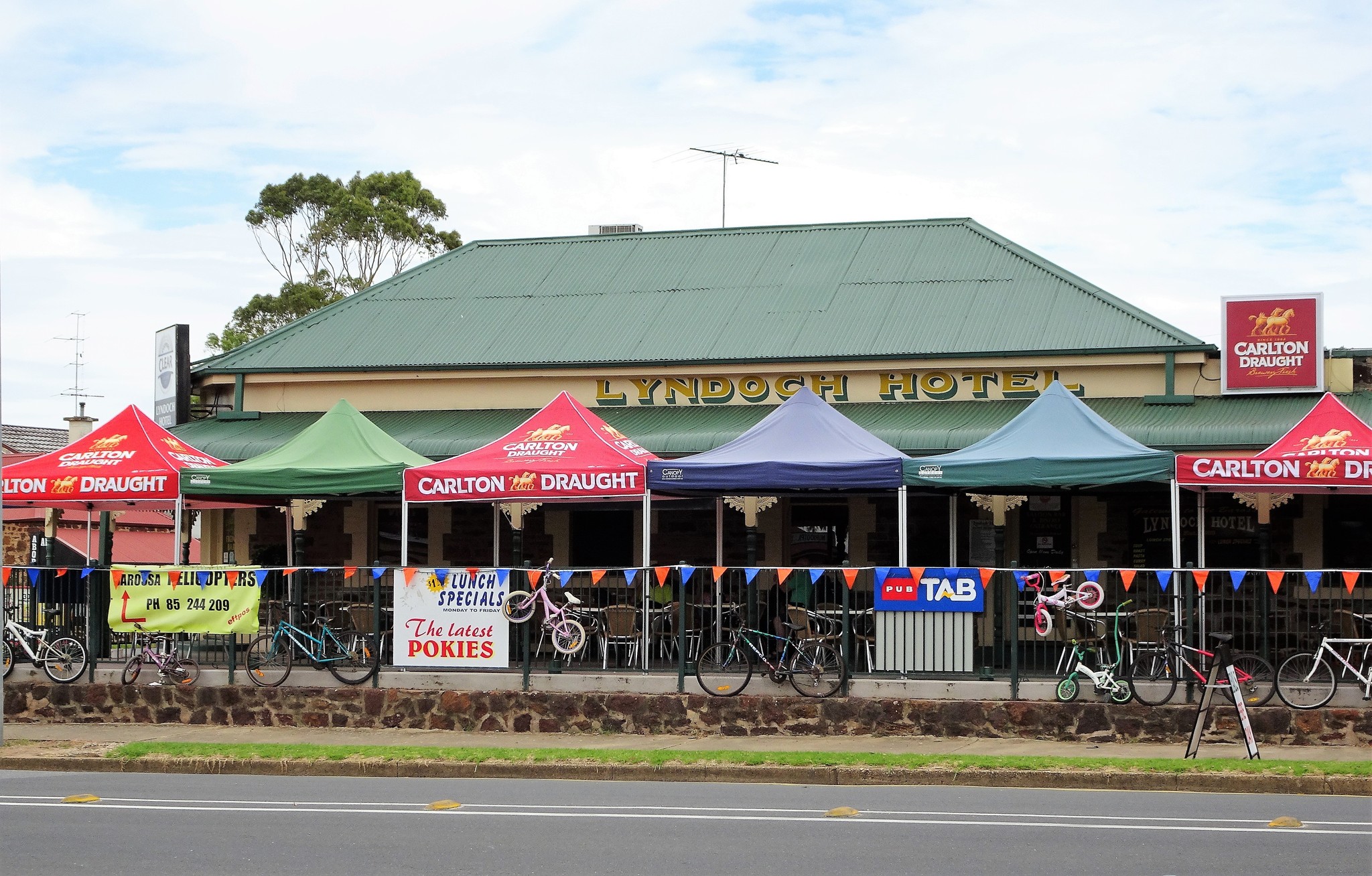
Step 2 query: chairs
1053,603,1371,684
258,599,395,666
535,597,875,678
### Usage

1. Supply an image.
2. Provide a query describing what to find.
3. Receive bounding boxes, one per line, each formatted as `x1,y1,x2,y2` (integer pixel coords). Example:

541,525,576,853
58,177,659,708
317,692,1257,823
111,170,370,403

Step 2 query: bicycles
501,556,587,654
245,594,377,689
695,602,846,698
1055,638,1135,705
1275,619,1372,710
3,604,87,683
1127,620,1275,706
1021,567,1104,638
121,621,200,686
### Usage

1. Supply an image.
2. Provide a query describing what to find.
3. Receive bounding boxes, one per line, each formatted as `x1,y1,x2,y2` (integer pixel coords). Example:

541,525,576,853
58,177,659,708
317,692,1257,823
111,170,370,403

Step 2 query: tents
901,379,1177,568
647,386,908,568
181,396,448,569
2,403,285,566
1176,387,1372,569
403,389,663,566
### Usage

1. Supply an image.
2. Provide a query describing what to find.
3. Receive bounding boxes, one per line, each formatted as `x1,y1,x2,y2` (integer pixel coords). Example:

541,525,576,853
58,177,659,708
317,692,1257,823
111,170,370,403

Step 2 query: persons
631,564,672,610
758,558,822,659
